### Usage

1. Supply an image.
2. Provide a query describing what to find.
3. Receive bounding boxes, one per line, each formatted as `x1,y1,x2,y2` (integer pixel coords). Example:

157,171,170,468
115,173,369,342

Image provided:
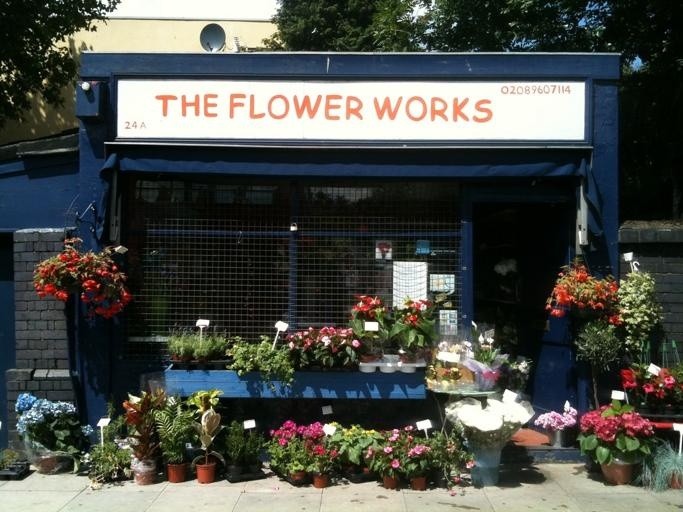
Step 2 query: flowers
16,236,683,496
